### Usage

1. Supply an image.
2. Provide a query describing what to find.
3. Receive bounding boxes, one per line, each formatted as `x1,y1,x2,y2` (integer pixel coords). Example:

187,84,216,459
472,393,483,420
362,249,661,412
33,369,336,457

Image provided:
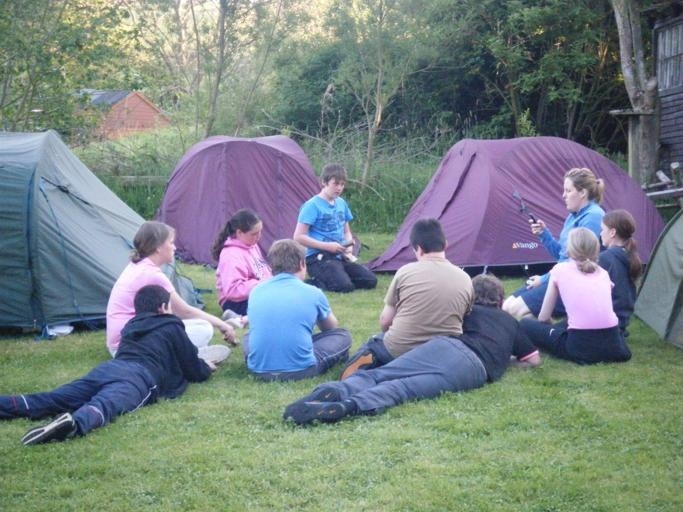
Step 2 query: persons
241,239,352,382
211,208,273,328
502,168,642,365
106,221,237,363
293,164,377,293
339,218,476,381
0,284,217,445
283,274,541,424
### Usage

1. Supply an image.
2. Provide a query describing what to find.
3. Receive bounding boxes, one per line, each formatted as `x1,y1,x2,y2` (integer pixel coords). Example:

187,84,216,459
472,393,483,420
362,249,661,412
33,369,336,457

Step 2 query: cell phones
528,213,537,223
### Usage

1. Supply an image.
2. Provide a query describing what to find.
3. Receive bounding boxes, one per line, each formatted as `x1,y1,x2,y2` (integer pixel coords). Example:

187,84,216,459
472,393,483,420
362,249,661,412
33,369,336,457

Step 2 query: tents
0,129,206,340
154,135,370,275
633,208,683,348
364,136,665,277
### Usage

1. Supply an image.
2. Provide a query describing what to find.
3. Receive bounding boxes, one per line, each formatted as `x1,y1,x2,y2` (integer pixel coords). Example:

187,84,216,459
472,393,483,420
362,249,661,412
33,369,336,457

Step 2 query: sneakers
339,350,376,381
222,309,241,329
198,345,230,364
283,387,352,422
21,412,75,445
